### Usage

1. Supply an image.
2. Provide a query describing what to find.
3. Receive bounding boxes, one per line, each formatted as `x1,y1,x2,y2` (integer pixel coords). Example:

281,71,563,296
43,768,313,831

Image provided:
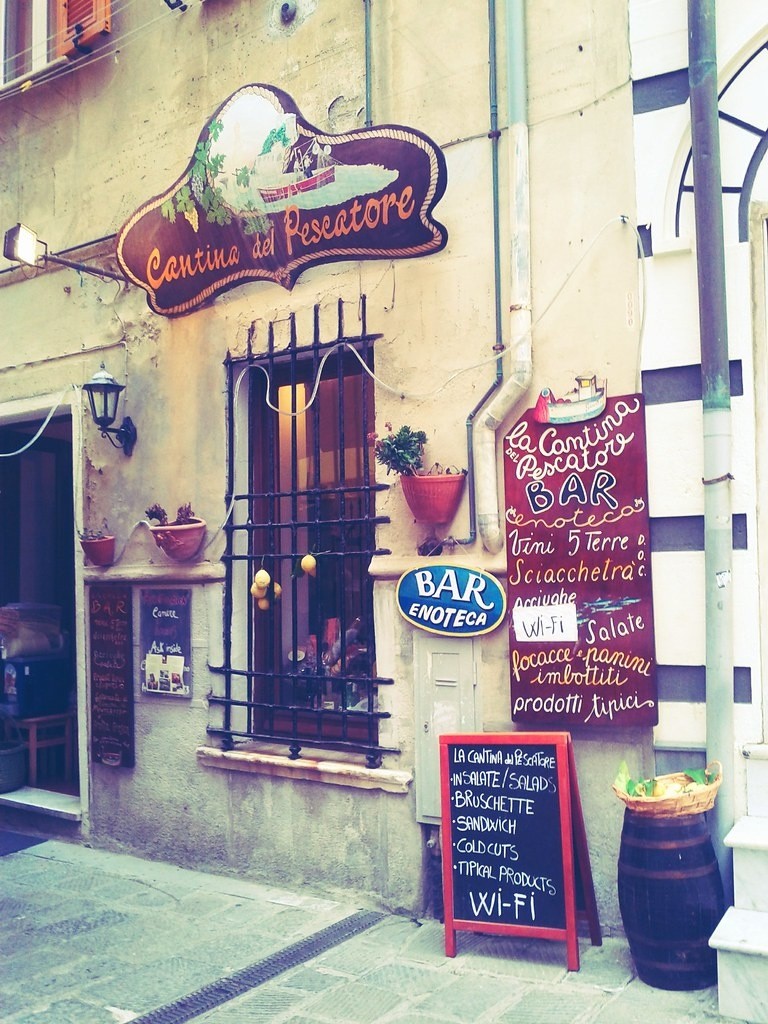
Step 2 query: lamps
84,364,138,461
4,224,134,293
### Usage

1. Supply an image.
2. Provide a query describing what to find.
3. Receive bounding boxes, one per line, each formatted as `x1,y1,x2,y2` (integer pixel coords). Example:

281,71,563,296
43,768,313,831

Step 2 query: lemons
250,555,316,610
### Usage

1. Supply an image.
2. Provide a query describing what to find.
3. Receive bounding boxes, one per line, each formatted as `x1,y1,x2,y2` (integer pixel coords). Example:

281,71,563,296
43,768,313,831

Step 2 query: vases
398,475,467,525
150,518,209,563
80,536,116,566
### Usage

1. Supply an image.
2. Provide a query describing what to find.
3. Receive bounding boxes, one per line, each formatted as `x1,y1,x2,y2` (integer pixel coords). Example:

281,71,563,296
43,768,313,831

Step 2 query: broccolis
626,768,715,797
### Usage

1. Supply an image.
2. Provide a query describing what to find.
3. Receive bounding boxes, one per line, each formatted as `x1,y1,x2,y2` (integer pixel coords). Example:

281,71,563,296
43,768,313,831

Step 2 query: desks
4,712,72,787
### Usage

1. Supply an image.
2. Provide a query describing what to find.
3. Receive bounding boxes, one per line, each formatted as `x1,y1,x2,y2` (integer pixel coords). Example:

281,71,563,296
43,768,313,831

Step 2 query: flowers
363,421,465,474
77,527,105,540
144,497,197,525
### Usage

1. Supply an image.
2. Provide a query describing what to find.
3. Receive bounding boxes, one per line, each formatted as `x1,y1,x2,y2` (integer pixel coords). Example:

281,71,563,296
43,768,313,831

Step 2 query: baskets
611,760,723,818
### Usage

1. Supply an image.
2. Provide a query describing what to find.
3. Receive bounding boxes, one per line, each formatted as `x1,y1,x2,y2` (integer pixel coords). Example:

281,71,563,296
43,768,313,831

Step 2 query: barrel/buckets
618,808,725,992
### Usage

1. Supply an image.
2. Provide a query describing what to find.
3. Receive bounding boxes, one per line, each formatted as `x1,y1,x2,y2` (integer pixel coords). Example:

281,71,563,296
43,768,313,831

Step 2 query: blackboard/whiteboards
89,586,135,766
140,587,194,697
438,731,574,937
